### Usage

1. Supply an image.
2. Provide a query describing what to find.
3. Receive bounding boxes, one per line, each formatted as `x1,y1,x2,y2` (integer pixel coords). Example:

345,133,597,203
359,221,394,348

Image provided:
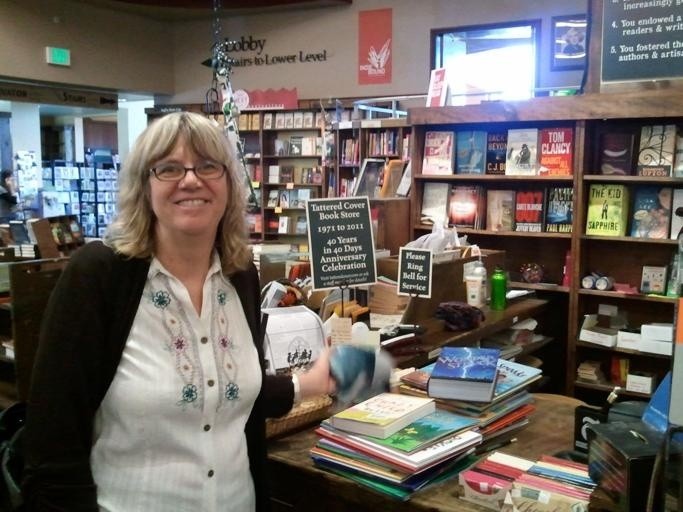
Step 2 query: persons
18,108,339,511
0,167,25,226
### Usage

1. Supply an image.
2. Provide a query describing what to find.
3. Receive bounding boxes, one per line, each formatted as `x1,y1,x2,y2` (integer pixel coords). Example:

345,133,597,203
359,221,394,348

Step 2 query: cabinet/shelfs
1,212,86,411
143,85,682,406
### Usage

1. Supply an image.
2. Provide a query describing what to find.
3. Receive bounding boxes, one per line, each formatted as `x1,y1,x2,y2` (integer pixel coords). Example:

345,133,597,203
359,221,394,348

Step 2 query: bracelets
290,372,301,404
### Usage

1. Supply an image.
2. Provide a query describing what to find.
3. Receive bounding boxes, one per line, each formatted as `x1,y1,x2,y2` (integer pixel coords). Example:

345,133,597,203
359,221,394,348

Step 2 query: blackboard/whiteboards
397,246,433,299
587,0,683,95
305,195,377,293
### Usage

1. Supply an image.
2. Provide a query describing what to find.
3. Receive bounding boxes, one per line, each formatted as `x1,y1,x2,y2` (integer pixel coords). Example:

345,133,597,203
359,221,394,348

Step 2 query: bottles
490,265,507,311
581,274,615,290
465,262,488,310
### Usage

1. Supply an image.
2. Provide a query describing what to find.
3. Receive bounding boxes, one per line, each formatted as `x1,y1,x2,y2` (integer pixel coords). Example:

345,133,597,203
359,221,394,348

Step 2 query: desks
250,386,682,512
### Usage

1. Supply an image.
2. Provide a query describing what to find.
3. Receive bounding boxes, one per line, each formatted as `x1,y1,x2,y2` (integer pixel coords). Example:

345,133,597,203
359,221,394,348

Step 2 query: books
225,108,405,242
33,162,118,239
4,242,40,260
639,265,668,296
418,120,682,242
366,273,411,318
424,65,449,108
572,304,678,398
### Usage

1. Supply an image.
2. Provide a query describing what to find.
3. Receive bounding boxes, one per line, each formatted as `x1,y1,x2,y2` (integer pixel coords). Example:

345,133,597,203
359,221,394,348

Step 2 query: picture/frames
548,12,587,71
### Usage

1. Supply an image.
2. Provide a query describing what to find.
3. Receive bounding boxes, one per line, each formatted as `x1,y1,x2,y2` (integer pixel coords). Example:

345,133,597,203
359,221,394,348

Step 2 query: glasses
144,160,226,182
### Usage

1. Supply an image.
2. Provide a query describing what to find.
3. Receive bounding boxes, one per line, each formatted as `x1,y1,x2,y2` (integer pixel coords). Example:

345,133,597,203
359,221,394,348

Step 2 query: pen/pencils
471,437,518,456
